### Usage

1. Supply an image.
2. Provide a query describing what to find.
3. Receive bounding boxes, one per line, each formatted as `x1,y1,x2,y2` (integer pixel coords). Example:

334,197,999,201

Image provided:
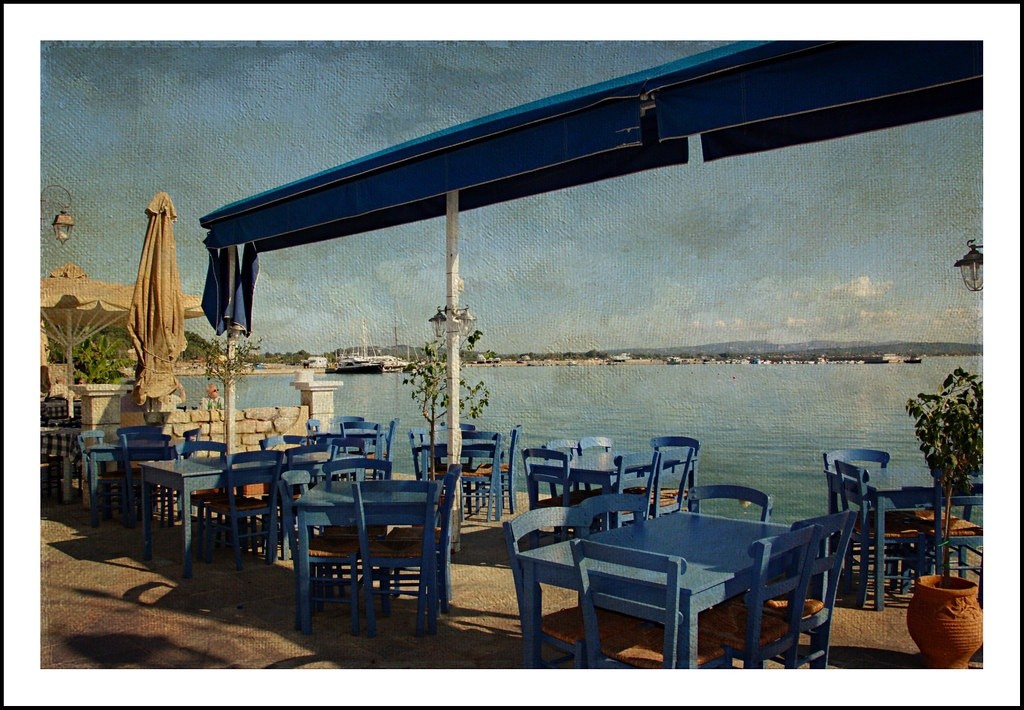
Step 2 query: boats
864,357,889,363
903,359,922,363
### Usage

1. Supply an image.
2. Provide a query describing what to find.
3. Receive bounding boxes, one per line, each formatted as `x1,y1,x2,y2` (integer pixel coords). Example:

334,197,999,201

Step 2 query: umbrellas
40,263,204,416
129,192,189,411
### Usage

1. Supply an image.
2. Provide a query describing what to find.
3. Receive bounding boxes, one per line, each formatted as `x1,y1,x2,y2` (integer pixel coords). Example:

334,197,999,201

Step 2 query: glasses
210,389,219,392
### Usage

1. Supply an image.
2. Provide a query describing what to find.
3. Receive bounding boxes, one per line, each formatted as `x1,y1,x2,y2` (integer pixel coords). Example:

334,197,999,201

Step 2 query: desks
137,456,287,578
530,450,699,548
86,440,174,528
292,480,446,634
823,464,983,612
313,429,384,438
40,400,82,504
431,438,505,522
516,511,834,669
262,452,365,579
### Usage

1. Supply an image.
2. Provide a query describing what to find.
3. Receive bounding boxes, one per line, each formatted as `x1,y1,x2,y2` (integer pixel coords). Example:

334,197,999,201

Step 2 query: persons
162,382,186,409
77,377,84,385
52,376,77,400
199,383,224,410
121,380,147,411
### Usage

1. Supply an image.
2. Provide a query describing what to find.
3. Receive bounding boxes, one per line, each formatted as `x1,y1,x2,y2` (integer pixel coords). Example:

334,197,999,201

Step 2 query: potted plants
903,366,983,669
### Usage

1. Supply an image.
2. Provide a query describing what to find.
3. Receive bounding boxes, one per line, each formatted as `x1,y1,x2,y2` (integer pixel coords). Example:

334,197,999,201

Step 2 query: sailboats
324,293,426,373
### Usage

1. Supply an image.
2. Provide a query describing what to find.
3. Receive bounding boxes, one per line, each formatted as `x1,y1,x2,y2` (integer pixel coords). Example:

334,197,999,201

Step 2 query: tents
204,41,983,472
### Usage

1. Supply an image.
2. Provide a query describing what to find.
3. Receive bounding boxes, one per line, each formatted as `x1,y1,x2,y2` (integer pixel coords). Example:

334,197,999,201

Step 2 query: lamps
428,305,477,336
41,183,75,245
954,238,983,291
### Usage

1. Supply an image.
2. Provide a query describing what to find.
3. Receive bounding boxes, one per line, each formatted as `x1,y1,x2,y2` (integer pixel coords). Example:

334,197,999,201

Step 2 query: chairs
78,416,984,669
